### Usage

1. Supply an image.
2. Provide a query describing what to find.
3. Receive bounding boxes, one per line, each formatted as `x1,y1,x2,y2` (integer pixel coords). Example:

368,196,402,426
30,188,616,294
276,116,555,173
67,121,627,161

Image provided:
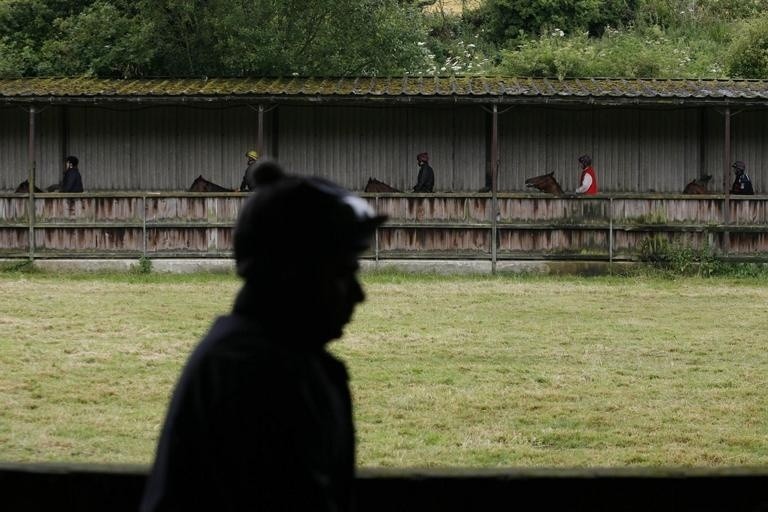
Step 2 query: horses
523,171,563,193
188,173,236,192
15,178,46,193
684,177,708,194
363,175,406,193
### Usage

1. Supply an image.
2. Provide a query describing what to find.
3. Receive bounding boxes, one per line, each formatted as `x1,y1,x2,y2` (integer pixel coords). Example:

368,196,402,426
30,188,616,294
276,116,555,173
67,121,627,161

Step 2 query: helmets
579,155,590,168
417,152,428,163
67,156,77,165
234,162,389,268
733,161,744,170
247,150,258,162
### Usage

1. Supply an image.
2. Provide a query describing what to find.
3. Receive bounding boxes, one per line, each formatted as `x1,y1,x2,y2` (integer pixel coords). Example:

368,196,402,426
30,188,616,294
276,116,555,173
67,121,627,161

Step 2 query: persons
48,156,84,194
234,149,258,193
137,157,391,509
572,155,597,194
728,160,755,196
409,152,435,193
